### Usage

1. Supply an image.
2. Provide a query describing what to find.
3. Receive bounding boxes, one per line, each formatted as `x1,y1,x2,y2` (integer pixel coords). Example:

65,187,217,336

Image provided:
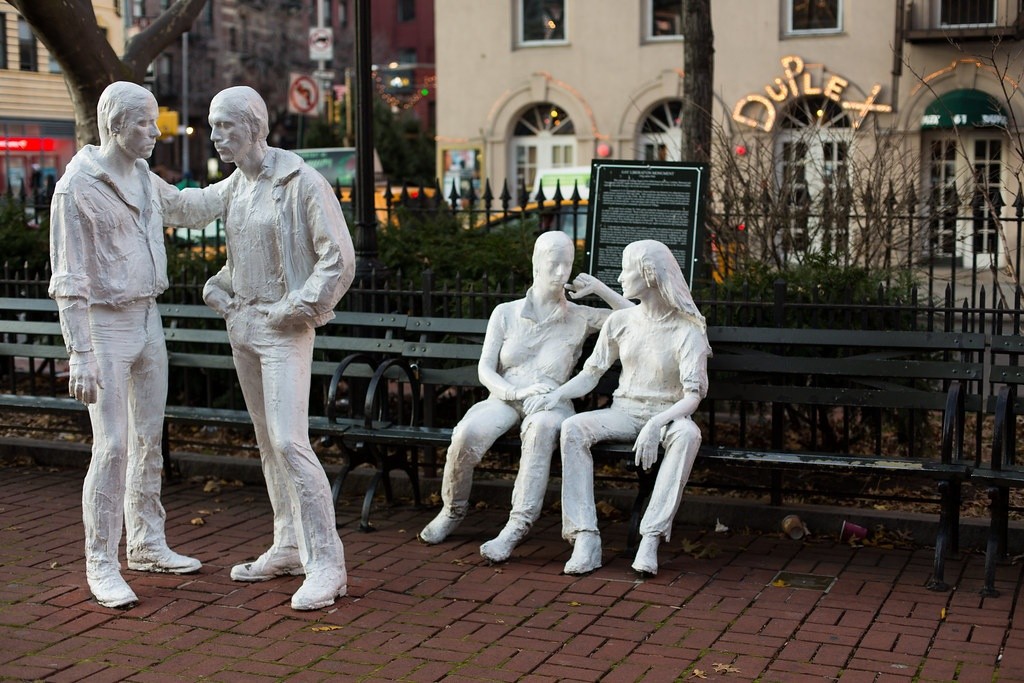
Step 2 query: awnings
921,87,1008,129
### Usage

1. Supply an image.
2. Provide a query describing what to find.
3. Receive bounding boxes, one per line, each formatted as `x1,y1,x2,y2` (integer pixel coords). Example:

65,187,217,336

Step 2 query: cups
838,521,867,546
782,516,804,539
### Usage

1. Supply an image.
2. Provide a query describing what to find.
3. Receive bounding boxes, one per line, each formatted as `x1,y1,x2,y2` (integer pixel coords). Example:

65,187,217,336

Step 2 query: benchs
969,334,1024,597
1,297,408,526
347,315,986,599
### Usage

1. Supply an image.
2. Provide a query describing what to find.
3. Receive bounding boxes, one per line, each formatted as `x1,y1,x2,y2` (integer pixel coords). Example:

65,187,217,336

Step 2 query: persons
47,80,239,609
419,230,639,564
202,84,358,610
520,238,713,575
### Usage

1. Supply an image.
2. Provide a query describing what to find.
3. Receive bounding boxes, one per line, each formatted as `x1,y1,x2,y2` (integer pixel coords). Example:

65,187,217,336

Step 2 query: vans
164,187,444,262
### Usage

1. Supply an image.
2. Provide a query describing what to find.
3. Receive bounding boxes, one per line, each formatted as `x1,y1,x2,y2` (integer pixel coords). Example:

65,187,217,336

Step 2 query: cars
461,198,749,284
